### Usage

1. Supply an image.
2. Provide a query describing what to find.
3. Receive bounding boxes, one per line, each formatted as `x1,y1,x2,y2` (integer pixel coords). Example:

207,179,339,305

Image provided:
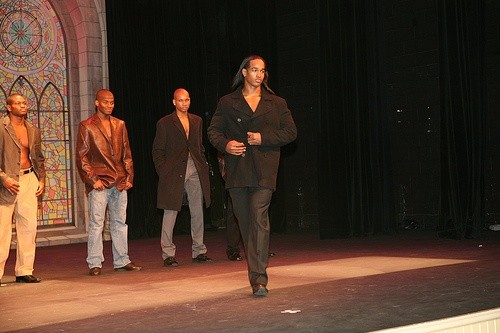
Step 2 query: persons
223,189,276,262
0,94,47,284
76,89,146,275
208,54,297,298
153,88,215,267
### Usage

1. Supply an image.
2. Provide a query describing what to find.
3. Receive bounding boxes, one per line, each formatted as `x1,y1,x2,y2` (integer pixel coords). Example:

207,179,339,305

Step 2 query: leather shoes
270,252,275,257
90,267,101,276
115,263,143,271
16,275,41,283
253,285,268,296
236,256,242,261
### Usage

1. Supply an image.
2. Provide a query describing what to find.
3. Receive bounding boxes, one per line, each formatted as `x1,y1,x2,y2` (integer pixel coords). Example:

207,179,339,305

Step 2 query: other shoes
192,254,213,264
164,257,178,267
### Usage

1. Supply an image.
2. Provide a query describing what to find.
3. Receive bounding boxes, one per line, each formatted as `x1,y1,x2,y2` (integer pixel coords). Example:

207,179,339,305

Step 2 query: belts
20,167,33,174
